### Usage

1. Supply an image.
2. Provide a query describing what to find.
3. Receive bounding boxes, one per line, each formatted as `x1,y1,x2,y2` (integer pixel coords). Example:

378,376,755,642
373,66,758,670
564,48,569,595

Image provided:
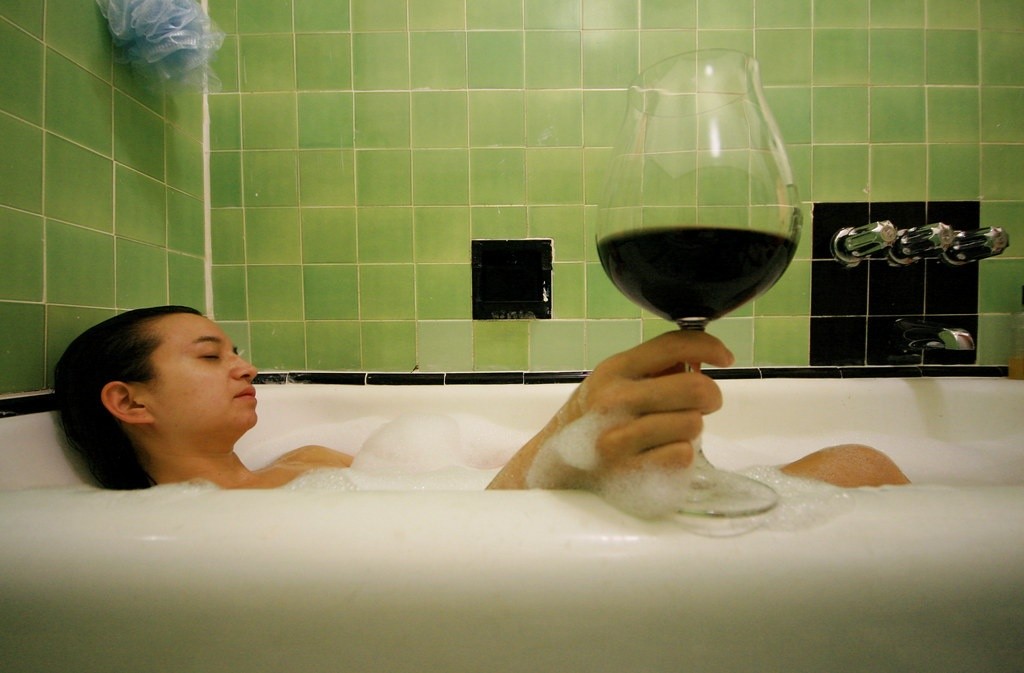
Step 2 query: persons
52,306,912,493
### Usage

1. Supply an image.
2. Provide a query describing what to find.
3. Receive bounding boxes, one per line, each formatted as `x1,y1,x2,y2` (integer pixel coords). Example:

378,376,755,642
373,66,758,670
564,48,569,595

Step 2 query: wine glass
596,47,804,518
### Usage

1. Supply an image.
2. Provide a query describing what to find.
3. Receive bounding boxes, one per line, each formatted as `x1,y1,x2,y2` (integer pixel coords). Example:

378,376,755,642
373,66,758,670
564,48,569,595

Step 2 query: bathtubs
0,382,1022,669
